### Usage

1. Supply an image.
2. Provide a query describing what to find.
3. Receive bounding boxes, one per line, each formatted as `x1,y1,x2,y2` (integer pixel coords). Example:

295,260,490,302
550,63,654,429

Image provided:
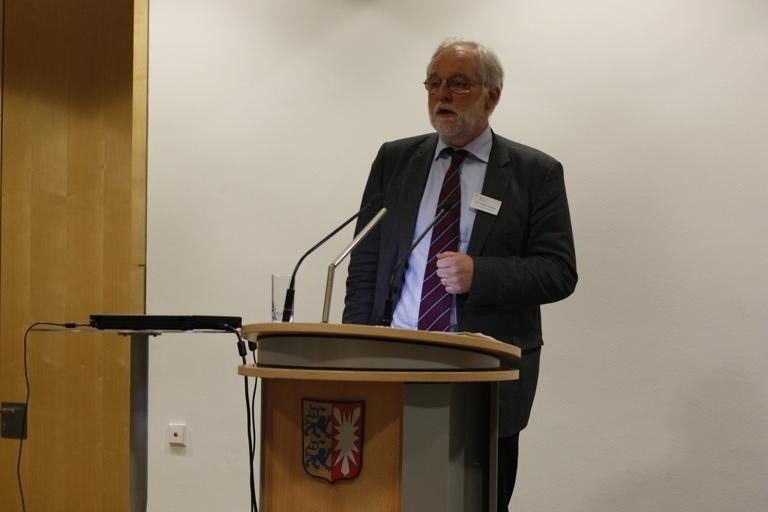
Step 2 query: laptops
89,314,242,330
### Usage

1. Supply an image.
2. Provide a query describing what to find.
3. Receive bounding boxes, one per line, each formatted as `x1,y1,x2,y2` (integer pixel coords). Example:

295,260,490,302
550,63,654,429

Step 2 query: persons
343,40,578,512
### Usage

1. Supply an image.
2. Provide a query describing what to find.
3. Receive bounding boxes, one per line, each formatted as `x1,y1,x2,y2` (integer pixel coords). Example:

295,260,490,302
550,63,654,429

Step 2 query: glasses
423,75,482,94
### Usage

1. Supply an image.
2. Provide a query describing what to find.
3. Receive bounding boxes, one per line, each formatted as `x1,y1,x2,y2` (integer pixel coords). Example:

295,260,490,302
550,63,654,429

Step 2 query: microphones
322,201,393,323
382,200,453,326
282,192,383,321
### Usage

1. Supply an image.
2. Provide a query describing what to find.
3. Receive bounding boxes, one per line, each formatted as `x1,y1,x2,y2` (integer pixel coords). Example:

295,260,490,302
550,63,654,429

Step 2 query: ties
418,146,470,332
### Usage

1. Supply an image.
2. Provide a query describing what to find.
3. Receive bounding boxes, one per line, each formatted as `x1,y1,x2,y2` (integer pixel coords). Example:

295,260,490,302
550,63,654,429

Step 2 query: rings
443,278,447,285
